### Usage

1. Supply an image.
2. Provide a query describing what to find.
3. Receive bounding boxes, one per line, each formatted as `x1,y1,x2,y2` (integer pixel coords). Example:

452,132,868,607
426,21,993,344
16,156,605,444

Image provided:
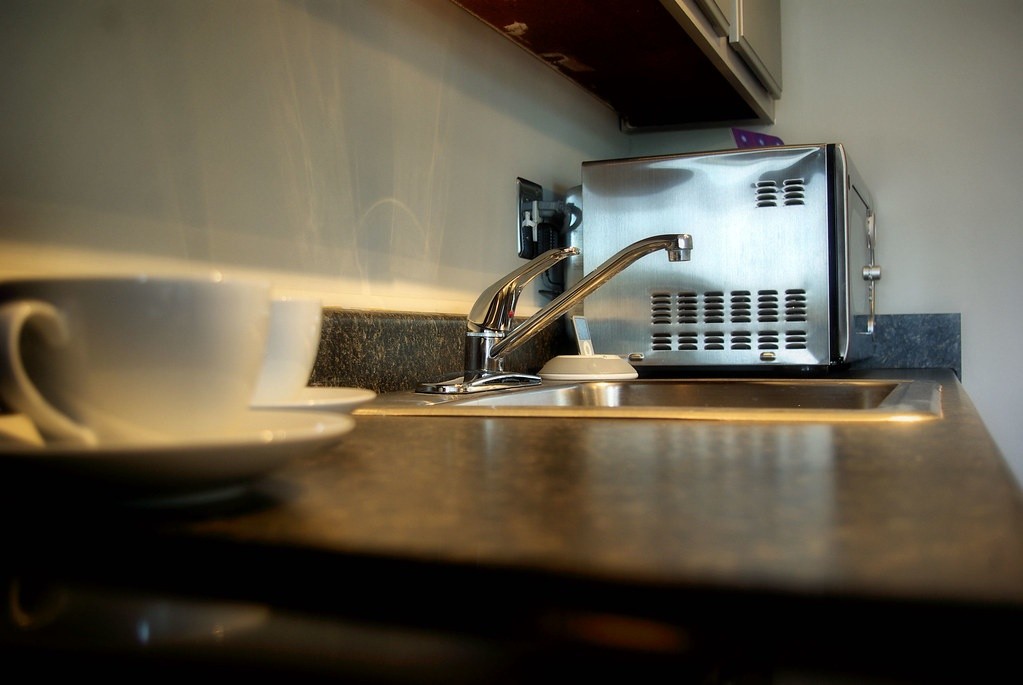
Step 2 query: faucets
461,233,692,376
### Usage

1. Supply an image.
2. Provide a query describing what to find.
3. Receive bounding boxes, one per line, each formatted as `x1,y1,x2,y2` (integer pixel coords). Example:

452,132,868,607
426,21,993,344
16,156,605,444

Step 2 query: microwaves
564,144,882,381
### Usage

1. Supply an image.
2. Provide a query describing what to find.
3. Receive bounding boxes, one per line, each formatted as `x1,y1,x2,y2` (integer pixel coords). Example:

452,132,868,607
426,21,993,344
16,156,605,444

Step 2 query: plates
0,412,355,479
252,387,378,413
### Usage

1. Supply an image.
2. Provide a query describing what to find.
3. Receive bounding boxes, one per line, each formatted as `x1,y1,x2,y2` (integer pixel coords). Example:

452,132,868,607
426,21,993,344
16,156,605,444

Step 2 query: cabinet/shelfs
453,0,782,138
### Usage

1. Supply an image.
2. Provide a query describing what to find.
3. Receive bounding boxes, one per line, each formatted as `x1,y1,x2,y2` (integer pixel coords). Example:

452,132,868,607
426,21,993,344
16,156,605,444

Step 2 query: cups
260,298,322,387
0,278,275,448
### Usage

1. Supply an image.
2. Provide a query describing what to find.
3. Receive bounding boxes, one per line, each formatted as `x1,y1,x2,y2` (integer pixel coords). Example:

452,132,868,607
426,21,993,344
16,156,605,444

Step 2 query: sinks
353,366,943,423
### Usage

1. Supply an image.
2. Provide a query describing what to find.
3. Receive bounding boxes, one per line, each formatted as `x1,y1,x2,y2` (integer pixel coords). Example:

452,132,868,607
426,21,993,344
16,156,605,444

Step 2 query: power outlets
516,178,542,259
